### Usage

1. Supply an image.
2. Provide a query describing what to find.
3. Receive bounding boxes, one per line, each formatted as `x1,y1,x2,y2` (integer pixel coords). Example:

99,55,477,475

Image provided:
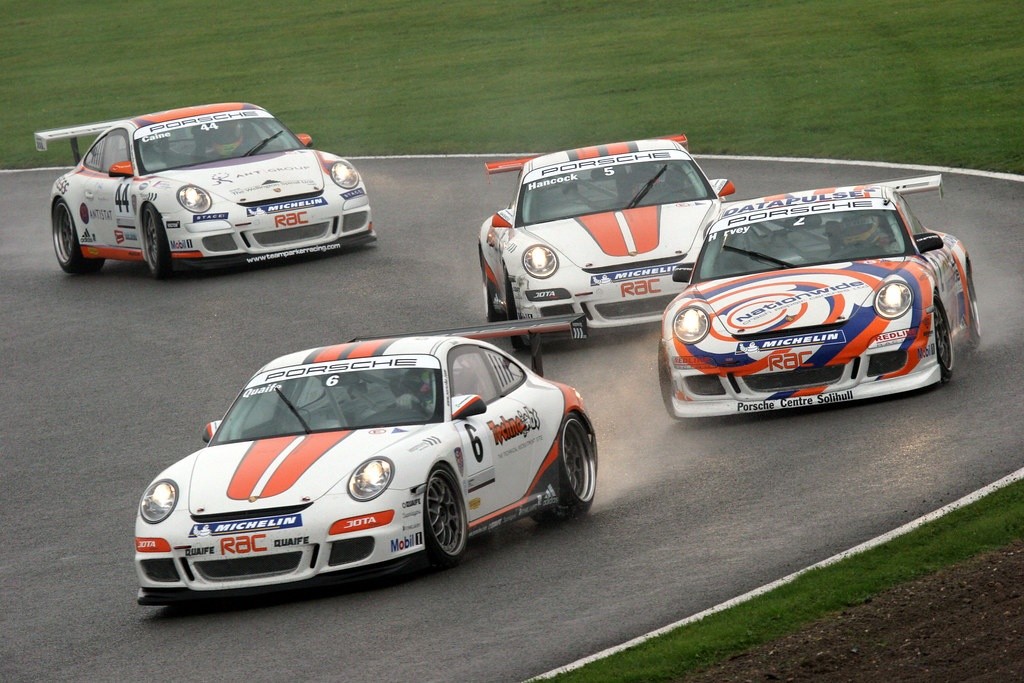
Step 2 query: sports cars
478,135,736,353
658,174,982,421
134,314,600,605
35,104,376,280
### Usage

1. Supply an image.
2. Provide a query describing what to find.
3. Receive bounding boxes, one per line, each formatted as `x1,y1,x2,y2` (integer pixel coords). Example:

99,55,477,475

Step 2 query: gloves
396,394,420,410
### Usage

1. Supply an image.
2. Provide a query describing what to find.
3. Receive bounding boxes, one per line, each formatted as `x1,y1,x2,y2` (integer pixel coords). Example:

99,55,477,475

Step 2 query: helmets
840,211,883,246
402,369,433,400
210,120,244,156
629,163,667,203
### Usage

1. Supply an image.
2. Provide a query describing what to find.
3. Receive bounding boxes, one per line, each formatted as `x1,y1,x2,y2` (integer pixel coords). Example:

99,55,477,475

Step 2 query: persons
209,121,245,159
827,210,899,251
385,369,433,413
634,163,665,195
